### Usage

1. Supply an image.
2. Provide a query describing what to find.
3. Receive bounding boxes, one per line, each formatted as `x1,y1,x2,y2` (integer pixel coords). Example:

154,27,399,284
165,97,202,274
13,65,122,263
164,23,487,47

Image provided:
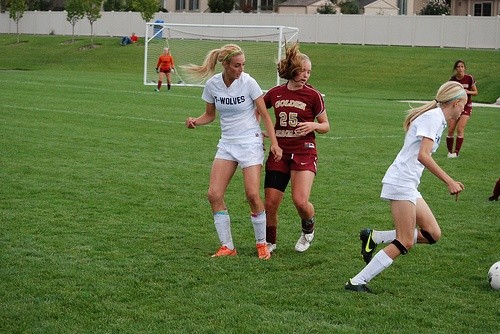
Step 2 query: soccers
488,261,500,290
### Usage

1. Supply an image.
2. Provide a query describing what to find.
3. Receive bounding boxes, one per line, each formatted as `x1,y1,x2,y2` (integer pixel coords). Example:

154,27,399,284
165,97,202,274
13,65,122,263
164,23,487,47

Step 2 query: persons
254,36,330,253
178,44,283,260
446,60,478,158
345,81,468,295
154,47,174,93
120,33,138,46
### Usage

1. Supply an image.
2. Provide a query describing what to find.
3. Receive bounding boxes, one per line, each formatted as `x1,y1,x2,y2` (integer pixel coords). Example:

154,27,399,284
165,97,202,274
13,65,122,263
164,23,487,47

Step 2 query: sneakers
360,229,377,265
447,152,460,158
211,246,236,258
345,278,373,294
295,230,314,252
256,243,271,260
268,243,276,252
